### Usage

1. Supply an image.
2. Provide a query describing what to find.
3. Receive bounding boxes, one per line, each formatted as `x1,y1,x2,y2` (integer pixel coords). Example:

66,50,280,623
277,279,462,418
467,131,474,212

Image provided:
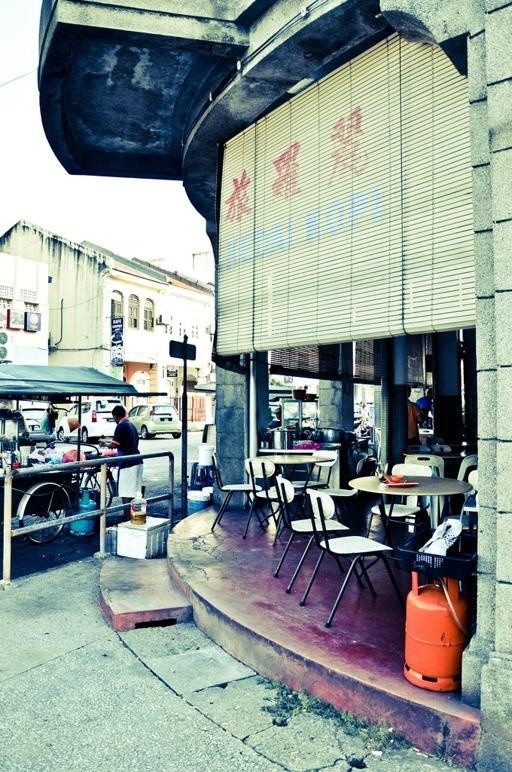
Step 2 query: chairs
405,452,476,581
242,456,302,540
360,463,435,565
307,426,379,480
290,449,338,530
271,473,350,578
285,487,406,628
212,449,270,533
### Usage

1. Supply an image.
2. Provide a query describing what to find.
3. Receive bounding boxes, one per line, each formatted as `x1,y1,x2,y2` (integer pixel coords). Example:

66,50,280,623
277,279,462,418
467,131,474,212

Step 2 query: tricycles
0,364,140,547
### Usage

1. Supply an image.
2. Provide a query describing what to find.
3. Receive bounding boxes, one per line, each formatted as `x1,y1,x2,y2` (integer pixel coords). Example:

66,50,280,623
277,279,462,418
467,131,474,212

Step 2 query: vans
55,397,125,443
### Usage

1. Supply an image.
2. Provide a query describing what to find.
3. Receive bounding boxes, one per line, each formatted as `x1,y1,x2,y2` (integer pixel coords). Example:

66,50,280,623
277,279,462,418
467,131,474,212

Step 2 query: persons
99,406,143,521
406,386,423,445
416,387,433,429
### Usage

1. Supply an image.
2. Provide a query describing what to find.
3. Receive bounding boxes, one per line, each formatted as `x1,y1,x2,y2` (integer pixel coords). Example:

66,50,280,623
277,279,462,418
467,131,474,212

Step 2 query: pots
318,426,342,442
272,429,296,449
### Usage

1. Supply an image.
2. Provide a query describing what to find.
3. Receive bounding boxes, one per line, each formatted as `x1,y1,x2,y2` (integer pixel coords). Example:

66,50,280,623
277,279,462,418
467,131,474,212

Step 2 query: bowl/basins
12,462,21,470
307,394,316,399
293,390,306,400
286,425,293,429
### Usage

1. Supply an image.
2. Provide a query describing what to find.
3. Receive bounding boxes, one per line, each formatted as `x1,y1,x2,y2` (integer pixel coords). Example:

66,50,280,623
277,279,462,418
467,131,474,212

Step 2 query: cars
282,400,374,429
127,403,182,440
20,404,53,435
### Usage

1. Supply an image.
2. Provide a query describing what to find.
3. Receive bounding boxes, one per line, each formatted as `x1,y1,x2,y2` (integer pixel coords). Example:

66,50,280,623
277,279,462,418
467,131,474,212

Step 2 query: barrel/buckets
199,446,214,465
187,491,210,517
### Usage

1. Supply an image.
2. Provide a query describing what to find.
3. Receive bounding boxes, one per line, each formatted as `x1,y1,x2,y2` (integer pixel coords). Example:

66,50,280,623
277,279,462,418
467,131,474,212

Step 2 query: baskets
397,540,477,581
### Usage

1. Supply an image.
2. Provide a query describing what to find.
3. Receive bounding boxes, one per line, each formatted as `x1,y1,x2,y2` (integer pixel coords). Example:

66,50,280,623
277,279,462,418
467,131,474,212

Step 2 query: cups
390,475,403,482
378,463,384,482
2,451,12,472
21,446,31,466
260,440,269,449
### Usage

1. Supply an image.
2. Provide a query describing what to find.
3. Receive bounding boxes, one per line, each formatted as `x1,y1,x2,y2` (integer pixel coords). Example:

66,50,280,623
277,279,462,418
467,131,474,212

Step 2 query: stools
191,461,215,489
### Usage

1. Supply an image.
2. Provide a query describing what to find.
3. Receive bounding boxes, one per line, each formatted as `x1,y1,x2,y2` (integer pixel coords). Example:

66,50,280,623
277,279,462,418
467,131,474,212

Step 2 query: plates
385,482,419,487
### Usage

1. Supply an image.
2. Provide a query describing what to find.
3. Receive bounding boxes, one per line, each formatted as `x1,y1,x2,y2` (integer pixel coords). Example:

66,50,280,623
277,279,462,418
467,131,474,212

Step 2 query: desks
347,475,474,583
258,453,335,540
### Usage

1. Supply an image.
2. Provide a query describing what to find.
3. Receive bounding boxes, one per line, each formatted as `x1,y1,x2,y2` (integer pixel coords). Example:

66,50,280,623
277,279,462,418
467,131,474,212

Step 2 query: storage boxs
116,515,171,560
